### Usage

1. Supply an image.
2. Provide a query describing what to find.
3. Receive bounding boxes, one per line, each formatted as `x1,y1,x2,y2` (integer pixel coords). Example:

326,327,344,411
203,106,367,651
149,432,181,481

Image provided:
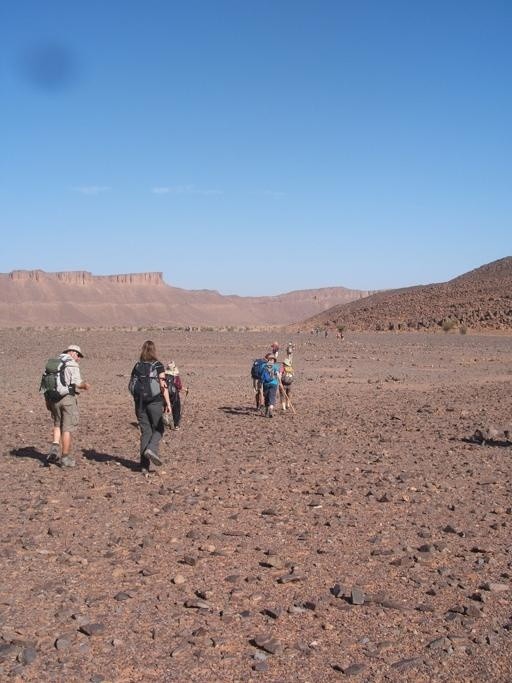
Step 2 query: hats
268,355,277,363
283,359,289,366
62,345,84,358
164,360,179,377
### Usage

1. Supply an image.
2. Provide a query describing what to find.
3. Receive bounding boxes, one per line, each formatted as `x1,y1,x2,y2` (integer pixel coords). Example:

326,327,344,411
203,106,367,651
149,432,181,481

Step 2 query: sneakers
47,452,75,468
174,425,181,431
141,449,163,472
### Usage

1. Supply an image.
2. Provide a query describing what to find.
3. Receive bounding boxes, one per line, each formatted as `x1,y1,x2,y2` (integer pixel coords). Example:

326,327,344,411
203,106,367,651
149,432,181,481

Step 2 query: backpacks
165,374,178,403
130,359,161,402
281,367,294,385
41,354,74,403
251,358,275,382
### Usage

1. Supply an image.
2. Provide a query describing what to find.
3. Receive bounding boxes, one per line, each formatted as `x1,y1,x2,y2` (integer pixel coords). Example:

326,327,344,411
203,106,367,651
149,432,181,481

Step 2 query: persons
271,341,279,357
164,361,187,430
260,354,283,418
250,351,272,408
280,358,293,411
127,340,174,477
286,342,294,358
44,341,89,467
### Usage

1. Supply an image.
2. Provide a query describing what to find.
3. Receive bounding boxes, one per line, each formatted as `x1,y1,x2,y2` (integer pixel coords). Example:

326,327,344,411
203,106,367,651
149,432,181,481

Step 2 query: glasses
75,352,80,357
